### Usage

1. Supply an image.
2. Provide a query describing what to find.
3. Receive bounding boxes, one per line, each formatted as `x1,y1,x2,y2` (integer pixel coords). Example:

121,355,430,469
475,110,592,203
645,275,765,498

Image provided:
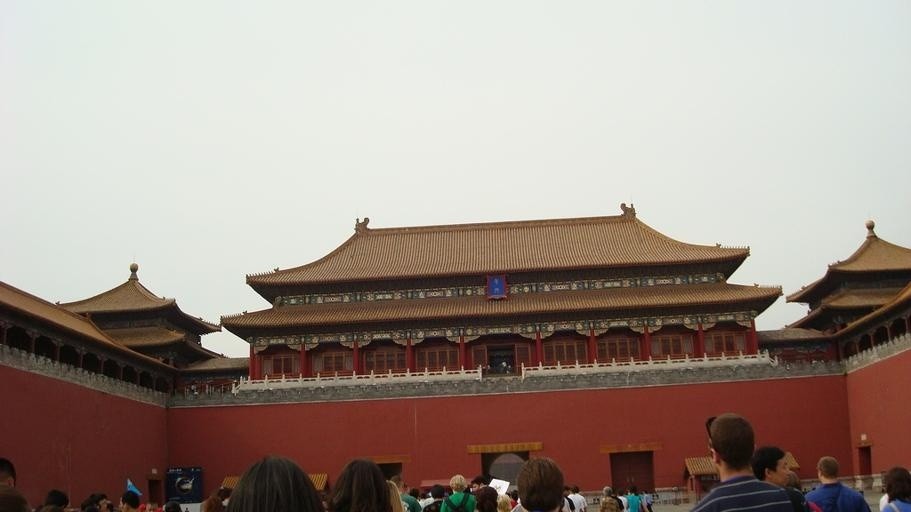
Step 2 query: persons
1,458,183,512
880,467,910,512
599,486,654,512
516,456,587,512
198,455,527,512
691,413,873,512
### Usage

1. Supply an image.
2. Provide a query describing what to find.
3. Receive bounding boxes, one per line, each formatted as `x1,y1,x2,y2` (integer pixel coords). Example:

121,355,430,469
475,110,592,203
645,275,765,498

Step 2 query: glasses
706,416,717,442
